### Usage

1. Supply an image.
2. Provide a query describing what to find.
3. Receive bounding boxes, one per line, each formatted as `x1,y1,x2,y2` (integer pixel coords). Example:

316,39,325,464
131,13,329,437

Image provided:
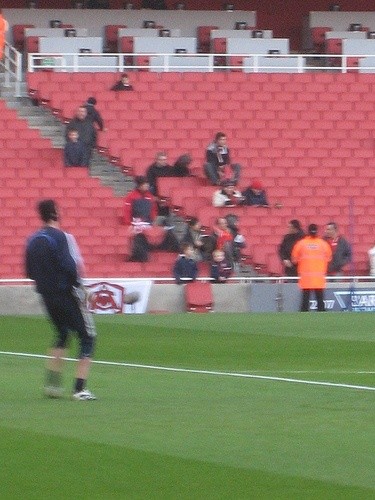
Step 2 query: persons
291,224,333,312
367,245,375,282
81,97,103,131
321,221,351,282
126,176,157,262
110,73,134,91
210,250,233,283
146,152,247,269
65,106,97,152
242,183,268,204
64,129,90,166
24,199,98,401
173,242,199,283
203,132,240,185
279,220,306,283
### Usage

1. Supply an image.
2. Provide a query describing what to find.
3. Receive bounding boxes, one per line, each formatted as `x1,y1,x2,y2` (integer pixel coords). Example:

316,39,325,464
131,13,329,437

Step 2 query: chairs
0,73,375,283
184,283,214,312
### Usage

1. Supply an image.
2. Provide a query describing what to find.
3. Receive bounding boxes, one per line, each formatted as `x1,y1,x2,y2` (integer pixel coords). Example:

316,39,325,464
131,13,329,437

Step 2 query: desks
199,26,306,74
309,10,375,74
105,25,209,73
13,24,119,73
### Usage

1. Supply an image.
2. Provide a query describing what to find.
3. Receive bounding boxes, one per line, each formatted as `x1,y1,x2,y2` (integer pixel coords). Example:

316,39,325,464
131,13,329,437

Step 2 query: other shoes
71,389,97,401
